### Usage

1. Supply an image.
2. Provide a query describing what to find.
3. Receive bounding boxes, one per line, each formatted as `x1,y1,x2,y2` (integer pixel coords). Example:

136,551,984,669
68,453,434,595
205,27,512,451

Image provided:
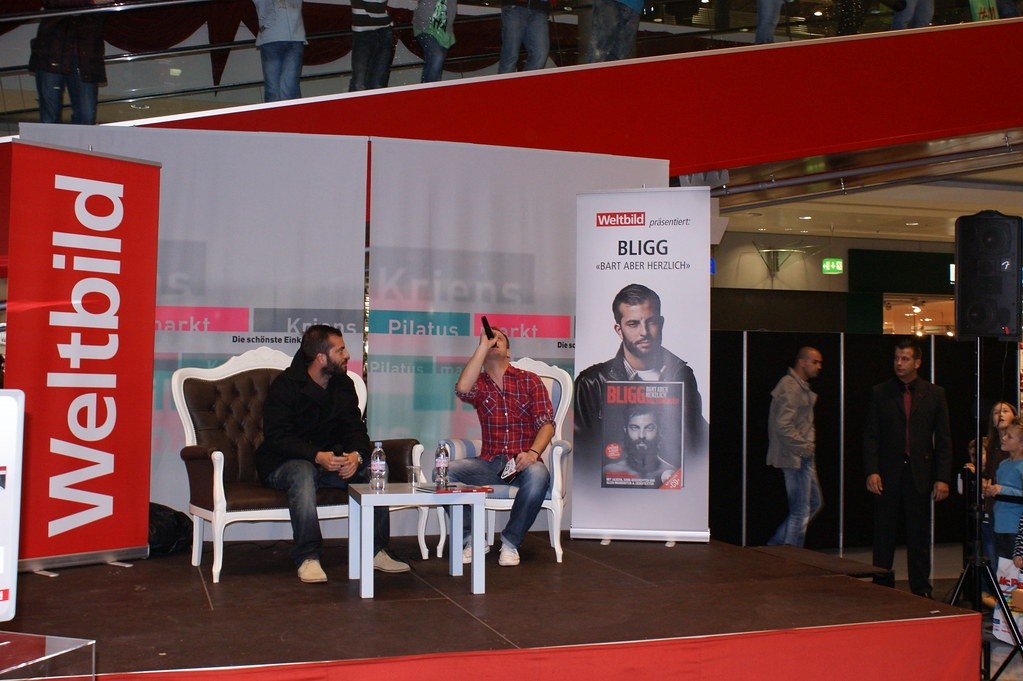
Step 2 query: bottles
434,440,449,489
370,441,387,491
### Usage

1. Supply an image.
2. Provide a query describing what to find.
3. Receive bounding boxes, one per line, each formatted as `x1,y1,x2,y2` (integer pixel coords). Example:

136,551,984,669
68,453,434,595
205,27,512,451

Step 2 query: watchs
353,451,364,465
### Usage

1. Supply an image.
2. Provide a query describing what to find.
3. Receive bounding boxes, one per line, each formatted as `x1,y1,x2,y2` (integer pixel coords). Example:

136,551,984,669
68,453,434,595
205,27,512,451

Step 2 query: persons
967,402,1023,607
27,0,114,126
857,339,953,598
588,0,645,67
347,0,398,92
439,327,556,566
411,0,460,82
253,0,308,103
891,0,935,33
573,284,709,491
603,406,682,488
260,325,411,583
765,346,823,547
498,0,550,73
754,0,784,45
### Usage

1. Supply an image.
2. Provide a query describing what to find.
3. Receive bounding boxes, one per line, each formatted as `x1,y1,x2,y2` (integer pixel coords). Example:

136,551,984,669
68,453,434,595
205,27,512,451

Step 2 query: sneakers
374,549,410,573
462,535,490,564
297,559,328,583
498,543,520,567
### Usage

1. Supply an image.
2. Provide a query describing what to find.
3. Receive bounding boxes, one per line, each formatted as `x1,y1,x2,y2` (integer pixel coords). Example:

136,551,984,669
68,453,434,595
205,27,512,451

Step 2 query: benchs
171,346,431,583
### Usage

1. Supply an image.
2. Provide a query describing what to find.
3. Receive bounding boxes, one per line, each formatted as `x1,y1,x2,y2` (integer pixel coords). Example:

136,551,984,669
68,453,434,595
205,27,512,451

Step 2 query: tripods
949,337,1023,681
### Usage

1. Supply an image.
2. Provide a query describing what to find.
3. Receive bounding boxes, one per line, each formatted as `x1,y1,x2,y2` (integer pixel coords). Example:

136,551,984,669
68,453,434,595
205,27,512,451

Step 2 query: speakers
954,209,1023,337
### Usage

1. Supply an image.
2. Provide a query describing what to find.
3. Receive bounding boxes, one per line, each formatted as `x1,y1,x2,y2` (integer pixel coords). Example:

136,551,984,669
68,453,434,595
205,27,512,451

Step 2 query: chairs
435,358,573,563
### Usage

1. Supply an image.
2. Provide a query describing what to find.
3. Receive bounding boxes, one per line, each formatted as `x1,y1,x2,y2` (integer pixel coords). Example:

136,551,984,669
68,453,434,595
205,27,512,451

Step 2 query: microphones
333,444,343,468
482,316,497,347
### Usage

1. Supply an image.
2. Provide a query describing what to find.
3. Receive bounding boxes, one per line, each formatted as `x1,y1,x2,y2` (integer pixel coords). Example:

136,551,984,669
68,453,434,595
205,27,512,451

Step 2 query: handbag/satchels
992,557,1023,650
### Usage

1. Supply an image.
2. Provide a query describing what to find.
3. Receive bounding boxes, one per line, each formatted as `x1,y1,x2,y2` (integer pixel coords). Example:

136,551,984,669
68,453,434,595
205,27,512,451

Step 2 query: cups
405,466,422,488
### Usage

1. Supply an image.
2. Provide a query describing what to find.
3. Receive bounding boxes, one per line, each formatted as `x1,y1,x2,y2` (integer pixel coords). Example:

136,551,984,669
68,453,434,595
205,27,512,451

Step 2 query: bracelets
529,448,540,456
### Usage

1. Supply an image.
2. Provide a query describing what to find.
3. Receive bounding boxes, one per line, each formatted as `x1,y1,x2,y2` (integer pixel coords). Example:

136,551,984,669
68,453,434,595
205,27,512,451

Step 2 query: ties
903,383,912,458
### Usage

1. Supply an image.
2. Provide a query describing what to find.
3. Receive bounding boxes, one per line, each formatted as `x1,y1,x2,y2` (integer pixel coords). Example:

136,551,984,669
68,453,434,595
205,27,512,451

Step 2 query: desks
348,484,486,599
